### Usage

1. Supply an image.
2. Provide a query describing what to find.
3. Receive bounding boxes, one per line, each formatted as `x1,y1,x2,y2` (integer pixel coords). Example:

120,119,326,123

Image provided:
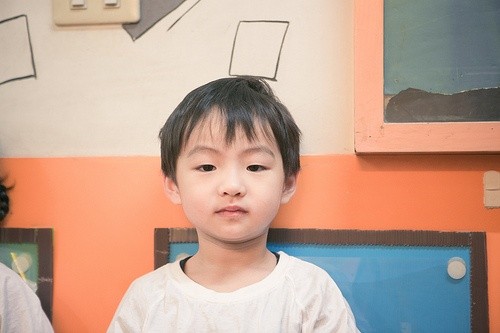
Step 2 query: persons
104,76,361,333
0,174,55,333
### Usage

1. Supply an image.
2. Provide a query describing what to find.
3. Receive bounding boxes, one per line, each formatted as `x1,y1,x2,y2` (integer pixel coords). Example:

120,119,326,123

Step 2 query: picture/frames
352,0,500,155
154,227,489,333
0,228,53,324
